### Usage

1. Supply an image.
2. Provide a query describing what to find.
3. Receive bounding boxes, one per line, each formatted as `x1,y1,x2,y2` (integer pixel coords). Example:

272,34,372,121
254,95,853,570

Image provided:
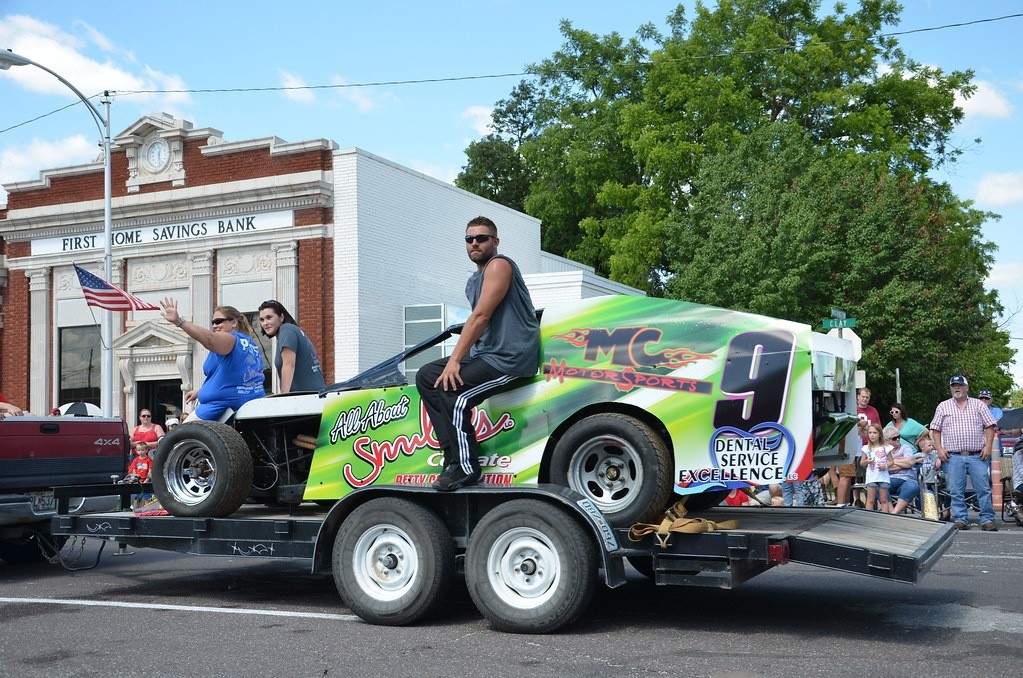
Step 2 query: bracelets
175,317,184,326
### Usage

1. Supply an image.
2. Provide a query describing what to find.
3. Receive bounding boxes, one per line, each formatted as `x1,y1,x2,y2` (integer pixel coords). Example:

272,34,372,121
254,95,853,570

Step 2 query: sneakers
432,471,486,490
954,522,968,530
982,523,998,530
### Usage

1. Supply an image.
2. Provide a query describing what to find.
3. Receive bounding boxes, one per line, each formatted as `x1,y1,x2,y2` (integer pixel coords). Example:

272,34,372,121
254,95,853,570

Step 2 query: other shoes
1015,513,1023,526
943,512,952,520
825,499,839,507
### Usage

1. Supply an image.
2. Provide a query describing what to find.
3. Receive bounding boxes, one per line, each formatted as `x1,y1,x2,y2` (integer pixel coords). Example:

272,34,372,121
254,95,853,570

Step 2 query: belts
948,450,982,456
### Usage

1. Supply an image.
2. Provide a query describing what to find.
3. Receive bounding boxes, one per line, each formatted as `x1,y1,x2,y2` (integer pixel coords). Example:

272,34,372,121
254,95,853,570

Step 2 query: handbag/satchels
262,368,273,389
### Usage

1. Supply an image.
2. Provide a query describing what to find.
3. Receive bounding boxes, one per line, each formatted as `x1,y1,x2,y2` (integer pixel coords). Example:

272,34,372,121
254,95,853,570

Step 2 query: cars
151,308,861,529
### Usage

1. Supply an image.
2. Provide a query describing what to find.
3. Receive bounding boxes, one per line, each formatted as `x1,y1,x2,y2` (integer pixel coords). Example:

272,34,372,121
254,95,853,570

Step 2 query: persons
128,408,189,506
718,383,1023,516
415,216,541,491
258,299,327,394
929,375,1000,531
48,408,61,416
0,394,24,420
160,296,265,424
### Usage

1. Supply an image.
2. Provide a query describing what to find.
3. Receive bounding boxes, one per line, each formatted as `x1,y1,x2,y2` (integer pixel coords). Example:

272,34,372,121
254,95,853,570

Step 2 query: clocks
147,142,166,167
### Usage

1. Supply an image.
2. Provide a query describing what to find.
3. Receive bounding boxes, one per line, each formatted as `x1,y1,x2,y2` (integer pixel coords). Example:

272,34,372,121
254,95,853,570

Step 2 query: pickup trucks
0,418,130,560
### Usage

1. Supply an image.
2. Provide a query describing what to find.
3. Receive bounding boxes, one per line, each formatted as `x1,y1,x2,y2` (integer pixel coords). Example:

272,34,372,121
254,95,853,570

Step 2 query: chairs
934,475,981,520
999,456,1023,523
876,464,924,517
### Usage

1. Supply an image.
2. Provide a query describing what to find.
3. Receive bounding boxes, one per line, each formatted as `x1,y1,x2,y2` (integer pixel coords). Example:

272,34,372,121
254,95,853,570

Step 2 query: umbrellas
55,399,103,418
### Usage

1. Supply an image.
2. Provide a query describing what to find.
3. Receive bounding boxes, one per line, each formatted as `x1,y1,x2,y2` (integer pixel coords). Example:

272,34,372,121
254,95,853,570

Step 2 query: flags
73,263,160,311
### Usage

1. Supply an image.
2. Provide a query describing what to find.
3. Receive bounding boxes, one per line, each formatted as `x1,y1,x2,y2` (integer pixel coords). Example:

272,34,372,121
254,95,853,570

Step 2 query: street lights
0,48,116,418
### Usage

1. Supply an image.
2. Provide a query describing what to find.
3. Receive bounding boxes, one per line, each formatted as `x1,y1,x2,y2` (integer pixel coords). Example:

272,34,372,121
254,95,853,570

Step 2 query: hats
980,390,993,398
165,418,179,426
950,375,968,387
50,407,60,414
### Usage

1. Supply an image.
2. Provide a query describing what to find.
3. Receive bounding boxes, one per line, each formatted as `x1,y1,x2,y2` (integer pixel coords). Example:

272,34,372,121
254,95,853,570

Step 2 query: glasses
890,410,901,415
213,318,235,325
52,412,60,416
887,435,901,441
465,235,496,244
140,414,151,418
979,397,991,401
